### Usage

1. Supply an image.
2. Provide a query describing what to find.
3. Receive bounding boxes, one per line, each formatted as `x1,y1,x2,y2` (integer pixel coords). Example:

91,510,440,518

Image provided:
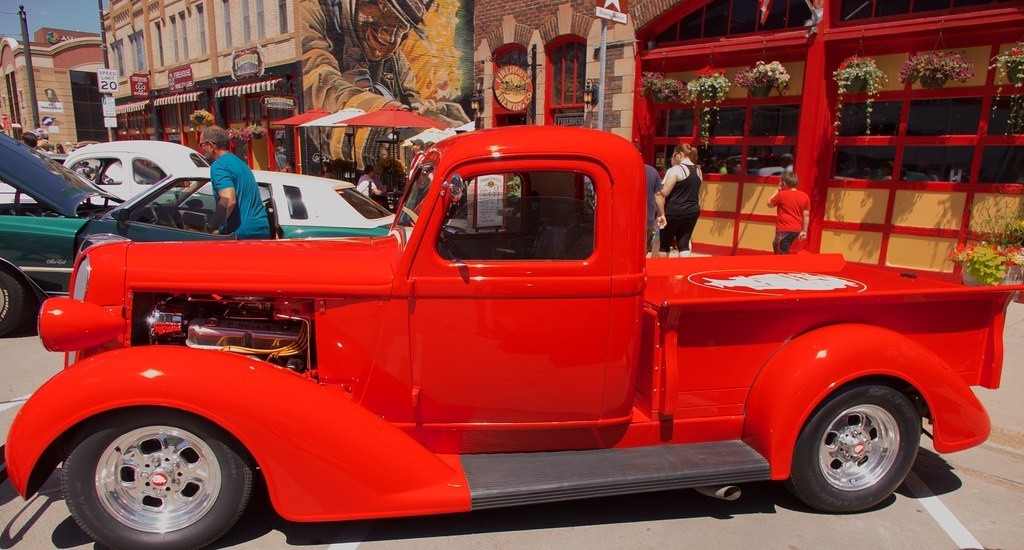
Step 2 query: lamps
583,78,599,108
123,125,196,136
471,88,485,113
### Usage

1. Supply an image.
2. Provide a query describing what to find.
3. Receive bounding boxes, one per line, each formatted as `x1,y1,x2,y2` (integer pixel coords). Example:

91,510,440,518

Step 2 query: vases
195,116,203,122
1007,61,1024,83
750,85,772,97
963,261,1024,302
699,86,717,100
253,133,261,140
845,74,867,93
652,89,670,102
920,74,948,89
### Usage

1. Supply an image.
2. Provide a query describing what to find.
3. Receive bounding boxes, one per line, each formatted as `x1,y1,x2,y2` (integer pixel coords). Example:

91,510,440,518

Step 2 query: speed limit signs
98,68,118,93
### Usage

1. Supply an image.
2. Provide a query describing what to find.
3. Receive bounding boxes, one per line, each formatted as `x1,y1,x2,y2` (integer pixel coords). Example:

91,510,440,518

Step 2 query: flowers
637,71,688,105
987,41,1024,138
946,195,1024,287
226,123,268,142
734,61,791,97
190,109,215,126
898,47,976,84
833,56,888,153
687,66,731,149
373,156,408,177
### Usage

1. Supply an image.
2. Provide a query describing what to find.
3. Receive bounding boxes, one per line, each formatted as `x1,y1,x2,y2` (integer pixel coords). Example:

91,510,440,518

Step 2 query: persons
38,140,67,155
199,126,271,240
357,139,434,201
658,143,704,257
277,147,286,173
21,132,48,157
633,143,667,258
768,171,811,254
704,152,907,183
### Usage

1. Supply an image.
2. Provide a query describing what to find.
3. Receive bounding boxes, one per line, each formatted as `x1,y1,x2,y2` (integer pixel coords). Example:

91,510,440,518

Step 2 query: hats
410,139,424,150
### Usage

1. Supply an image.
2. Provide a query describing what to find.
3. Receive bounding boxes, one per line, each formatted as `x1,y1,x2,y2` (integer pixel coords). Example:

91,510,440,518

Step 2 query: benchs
533,227,593,259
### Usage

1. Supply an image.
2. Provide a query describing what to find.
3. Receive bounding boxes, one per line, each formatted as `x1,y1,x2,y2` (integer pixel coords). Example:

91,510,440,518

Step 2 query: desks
387,192,404,208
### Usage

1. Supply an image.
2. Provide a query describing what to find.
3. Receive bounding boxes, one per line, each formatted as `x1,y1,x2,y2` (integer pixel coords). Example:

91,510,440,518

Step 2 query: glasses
198,141,217,149
673,152,680,158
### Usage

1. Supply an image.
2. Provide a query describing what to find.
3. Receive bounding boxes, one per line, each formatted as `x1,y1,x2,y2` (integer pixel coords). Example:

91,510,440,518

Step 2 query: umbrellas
274,107,452,190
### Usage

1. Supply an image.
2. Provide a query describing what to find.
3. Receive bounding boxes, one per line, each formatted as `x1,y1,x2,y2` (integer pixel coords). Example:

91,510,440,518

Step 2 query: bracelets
204,223,213,233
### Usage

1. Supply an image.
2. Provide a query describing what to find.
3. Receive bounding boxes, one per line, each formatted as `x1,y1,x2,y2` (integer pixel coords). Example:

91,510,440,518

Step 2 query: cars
172,167,395,230
0,134,394,339
58,140,220,207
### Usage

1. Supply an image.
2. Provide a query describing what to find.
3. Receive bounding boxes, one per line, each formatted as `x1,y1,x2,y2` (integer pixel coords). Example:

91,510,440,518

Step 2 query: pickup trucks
0,125,1023,550
